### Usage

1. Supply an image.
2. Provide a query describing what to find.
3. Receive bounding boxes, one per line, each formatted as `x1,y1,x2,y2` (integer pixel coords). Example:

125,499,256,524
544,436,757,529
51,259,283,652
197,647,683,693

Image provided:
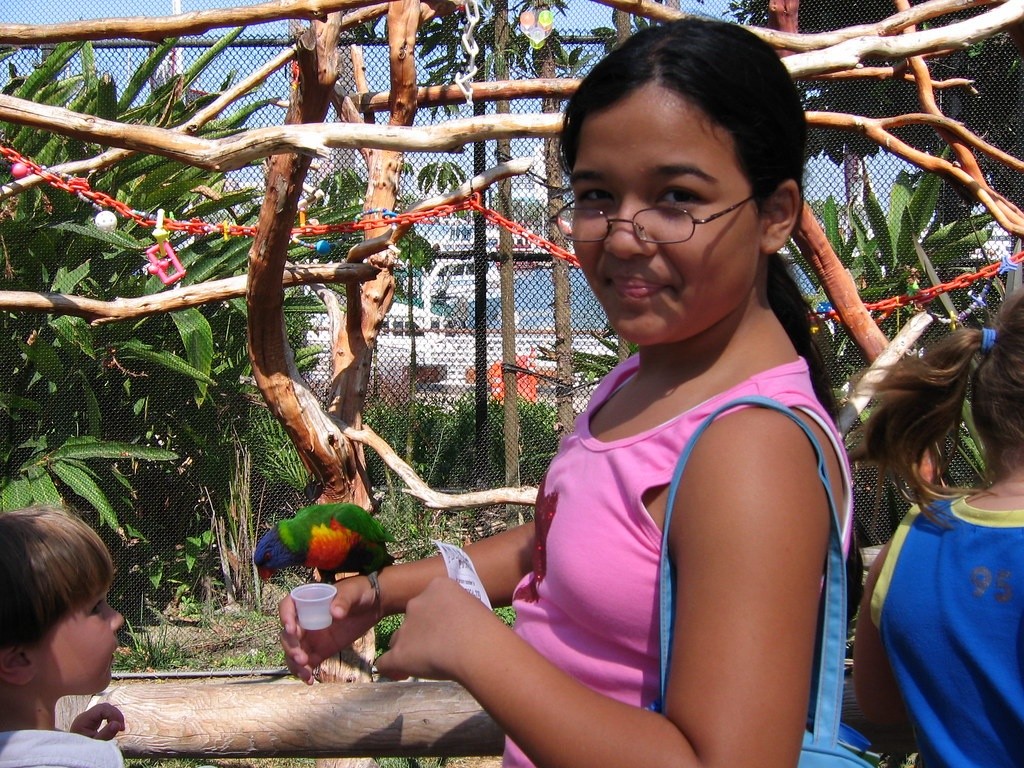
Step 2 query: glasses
548,195,755,244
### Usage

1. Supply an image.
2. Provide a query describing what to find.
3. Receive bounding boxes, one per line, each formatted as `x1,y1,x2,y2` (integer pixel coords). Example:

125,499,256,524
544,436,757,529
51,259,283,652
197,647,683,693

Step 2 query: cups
290,582,337,631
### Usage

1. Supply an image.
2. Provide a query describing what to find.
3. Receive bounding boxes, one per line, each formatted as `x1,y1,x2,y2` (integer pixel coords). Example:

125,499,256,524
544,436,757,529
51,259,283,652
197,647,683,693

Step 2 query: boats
413,251,503,308
306,303,444,354
970,224,1012,261
473,226,549,272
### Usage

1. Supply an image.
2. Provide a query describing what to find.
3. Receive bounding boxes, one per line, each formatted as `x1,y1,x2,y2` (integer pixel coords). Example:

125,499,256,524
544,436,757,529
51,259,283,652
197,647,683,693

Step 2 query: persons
278,14,851,768
0,504,126,768
854,291,1024,768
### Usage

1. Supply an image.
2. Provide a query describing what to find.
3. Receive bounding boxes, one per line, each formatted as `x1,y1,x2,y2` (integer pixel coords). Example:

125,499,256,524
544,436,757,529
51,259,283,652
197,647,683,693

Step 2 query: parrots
254,503,398,597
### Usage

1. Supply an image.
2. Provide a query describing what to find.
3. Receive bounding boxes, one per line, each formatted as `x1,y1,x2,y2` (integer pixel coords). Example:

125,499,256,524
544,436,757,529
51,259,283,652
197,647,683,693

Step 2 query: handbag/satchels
644,397,874,768
